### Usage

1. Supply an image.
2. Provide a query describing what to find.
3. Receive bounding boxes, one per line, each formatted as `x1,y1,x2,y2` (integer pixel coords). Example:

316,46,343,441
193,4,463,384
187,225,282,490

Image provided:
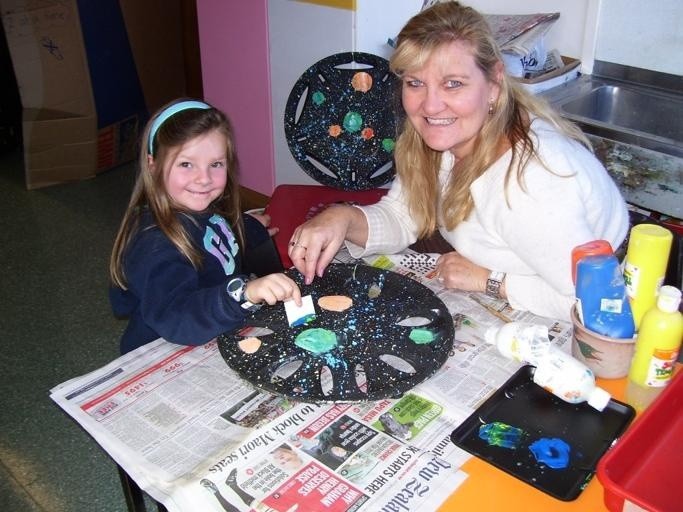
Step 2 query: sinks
561,83,683,144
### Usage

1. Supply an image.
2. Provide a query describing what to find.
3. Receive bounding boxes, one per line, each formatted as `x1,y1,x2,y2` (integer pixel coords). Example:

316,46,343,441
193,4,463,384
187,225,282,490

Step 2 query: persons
109,95,303,358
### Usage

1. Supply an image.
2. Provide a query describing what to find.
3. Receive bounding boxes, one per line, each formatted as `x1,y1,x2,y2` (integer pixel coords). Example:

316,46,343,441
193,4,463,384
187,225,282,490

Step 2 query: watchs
287,0,631,325
224,272,266,313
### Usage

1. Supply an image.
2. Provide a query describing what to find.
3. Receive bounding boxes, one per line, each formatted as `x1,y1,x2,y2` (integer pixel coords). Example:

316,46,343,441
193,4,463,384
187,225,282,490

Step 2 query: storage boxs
480,12,581,93
0,1,189,192
595,367,682,512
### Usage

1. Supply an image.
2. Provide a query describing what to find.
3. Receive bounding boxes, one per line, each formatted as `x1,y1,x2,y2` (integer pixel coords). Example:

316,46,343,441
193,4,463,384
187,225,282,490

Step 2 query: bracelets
485,270,505,297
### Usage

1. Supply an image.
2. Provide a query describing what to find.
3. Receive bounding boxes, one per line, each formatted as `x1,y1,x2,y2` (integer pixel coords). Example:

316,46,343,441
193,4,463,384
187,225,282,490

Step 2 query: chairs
255,49,410,269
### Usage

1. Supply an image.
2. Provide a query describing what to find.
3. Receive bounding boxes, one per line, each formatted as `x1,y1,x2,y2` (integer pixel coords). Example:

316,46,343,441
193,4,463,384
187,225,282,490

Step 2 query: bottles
568,239,635,342
531,345,610,415
481,321,549,360
622,223,673,332
625,284,682,389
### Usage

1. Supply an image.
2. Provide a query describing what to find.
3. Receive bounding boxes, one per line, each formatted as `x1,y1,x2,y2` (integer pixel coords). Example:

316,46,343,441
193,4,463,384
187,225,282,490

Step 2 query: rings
437,274,443,282
289,239,296,247
294,242,307,250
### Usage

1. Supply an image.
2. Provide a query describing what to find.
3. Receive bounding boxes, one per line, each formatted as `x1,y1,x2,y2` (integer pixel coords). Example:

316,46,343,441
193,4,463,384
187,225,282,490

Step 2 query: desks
48,243,682,512
